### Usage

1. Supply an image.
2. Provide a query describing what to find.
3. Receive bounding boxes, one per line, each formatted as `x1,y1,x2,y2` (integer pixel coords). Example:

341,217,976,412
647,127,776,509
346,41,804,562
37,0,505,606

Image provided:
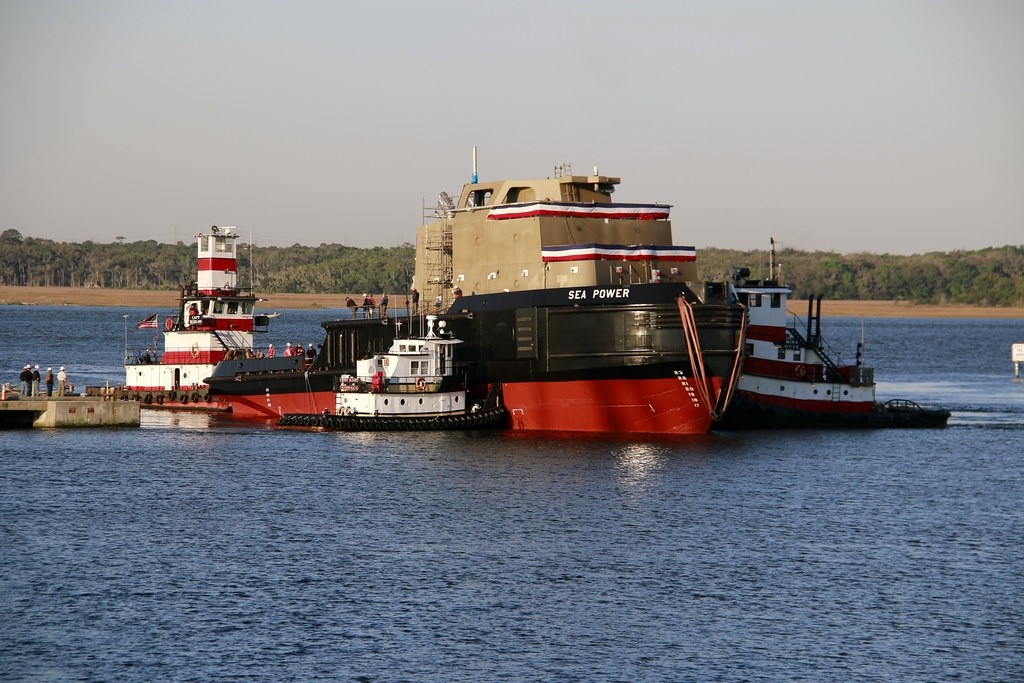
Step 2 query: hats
362,294,367,298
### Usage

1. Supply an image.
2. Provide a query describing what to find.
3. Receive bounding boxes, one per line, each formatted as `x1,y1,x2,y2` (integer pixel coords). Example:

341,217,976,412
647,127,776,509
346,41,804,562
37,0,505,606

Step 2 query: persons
453,285,462,299
346,297,358,319
267,344,275,358
144,348,151,363
379,294,388,318
294,342,305,362
245,349,254,359
412,289,419,312
256,348,264,359
45,366,54,397
20,364,33,396
31,365,41,397
318,345,323,350
306,344,316,366
283,343,295,357
188,304,197,330
223,349,238,361
57,366,67,397
362,293,375,319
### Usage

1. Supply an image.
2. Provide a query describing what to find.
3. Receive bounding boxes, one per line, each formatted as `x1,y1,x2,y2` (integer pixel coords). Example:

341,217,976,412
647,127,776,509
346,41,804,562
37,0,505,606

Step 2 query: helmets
413,287,417,291
27,364,31,368
47,367,53,371
35,365,40,369
309,343,313,347
268,344,273,348
23,367,27,369
287,342,291,347
453,285,459,290
60,366,65,371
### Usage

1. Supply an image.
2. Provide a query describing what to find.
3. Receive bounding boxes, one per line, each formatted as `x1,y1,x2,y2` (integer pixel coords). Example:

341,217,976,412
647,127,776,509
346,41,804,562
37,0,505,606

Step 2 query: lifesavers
131,394,139,401
179,394,187,404
145,394,152,403
278,413,499,430
120,395,126,401
168,391,174,400
203,393,211,402
191,342,199,356
191,392,198,402
416,378,426,390
157,395,163,403
165,318,174,330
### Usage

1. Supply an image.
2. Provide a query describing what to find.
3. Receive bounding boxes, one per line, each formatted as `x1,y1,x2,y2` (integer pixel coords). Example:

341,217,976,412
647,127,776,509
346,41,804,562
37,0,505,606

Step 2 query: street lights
122,314,128,364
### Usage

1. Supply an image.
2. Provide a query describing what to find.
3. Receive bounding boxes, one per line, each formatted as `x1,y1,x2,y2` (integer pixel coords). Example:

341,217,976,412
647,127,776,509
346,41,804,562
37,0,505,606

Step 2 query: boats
266,286,510,432
84,142,752,440
709,236,954,429
256,311,282,319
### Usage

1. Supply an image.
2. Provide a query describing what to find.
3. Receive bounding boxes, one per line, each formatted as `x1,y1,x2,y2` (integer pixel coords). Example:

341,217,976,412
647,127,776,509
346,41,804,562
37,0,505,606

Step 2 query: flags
137,313,157,328
